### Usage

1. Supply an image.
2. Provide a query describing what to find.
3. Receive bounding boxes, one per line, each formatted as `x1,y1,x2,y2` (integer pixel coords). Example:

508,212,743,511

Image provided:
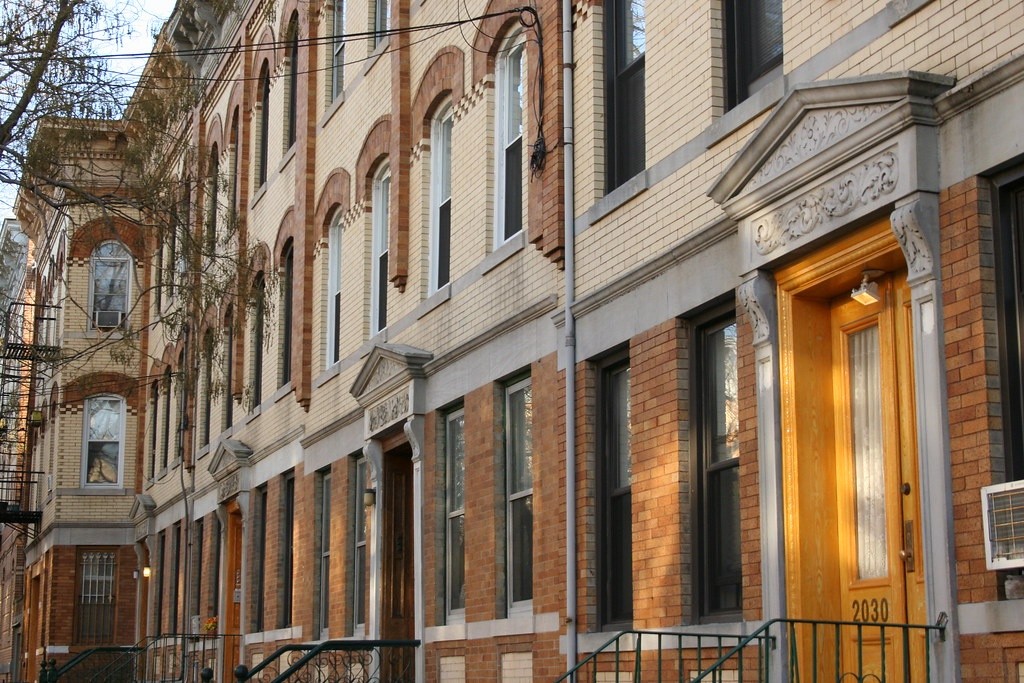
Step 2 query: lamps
363,488,376,506
30,410,42,427
850,269,885,306
132,570,139,578
143,564,150,577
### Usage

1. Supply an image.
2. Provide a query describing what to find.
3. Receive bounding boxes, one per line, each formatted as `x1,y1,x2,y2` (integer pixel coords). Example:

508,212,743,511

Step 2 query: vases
207,630,217,639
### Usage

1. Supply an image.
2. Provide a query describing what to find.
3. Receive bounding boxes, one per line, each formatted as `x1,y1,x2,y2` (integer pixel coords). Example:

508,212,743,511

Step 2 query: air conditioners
980,478,1024,572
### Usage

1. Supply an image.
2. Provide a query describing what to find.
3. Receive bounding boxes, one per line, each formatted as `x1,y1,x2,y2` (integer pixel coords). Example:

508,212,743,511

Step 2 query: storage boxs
95,311,121,327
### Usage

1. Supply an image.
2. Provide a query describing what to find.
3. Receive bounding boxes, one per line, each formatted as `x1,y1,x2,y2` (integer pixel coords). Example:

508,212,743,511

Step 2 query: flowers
202,618,217,631
